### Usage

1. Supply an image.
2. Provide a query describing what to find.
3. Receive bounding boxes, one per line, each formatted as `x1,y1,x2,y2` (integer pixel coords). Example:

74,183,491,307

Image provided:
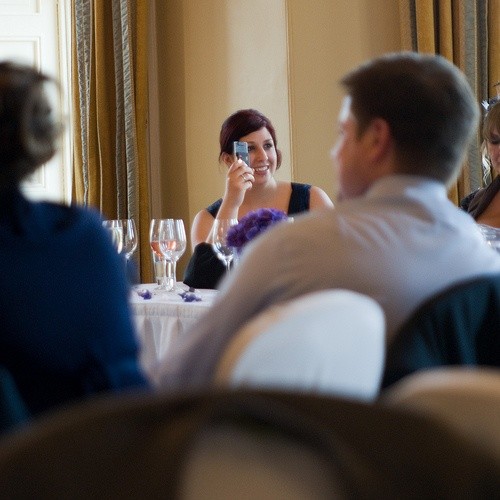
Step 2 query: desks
129,282,221,391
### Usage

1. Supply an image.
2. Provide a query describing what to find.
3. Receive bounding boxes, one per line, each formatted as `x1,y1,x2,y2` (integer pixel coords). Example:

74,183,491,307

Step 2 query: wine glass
102,219,138,278
149,217,187,292
212,217,240,276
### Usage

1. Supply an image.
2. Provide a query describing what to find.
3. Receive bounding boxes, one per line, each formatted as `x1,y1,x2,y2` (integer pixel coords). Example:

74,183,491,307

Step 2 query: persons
0,56,154,405
157,51,500,392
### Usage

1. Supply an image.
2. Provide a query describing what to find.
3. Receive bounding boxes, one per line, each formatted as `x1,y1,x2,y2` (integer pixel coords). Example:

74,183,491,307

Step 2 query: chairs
208,289,386,403
386,273,500,456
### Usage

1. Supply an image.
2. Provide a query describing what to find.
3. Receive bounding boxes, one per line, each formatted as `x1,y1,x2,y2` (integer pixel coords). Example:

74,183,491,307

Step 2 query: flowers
224,207,289,259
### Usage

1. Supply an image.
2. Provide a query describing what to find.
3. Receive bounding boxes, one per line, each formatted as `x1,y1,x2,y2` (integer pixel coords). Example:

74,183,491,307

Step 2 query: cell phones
234,141,250,168
